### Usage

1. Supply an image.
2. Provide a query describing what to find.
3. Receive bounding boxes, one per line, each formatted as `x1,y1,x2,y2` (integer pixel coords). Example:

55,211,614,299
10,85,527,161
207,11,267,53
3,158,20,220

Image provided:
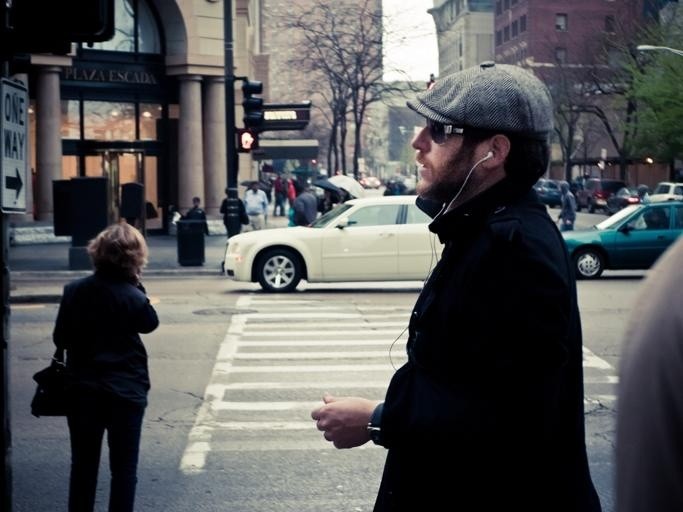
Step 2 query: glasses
426,118,466,143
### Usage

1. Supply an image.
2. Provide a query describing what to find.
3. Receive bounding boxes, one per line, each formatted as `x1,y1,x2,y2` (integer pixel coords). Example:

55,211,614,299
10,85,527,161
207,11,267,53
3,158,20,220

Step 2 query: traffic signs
0,77,29,215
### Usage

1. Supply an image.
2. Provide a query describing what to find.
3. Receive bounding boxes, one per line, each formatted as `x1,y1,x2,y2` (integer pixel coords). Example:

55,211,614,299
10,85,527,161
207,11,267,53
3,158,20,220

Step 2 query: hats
406,60,553,141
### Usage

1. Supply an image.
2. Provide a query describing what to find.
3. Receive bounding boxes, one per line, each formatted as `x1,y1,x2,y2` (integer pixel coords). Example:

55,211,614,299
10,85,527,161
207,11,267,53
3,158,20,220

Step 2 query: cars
360,176,382,190
532,178,562,208
221,192,450,294
606,184,654,216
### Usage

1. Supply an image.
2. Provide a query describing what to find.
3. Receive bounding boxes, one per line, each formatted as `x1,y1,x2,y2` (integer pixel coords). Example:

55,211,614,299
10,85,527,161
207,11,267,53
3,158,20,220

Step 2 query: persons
186,173,408,237
638,185,651,204
610,235,683,512
555,180,576,231
54,222,159,511
314,59,601,512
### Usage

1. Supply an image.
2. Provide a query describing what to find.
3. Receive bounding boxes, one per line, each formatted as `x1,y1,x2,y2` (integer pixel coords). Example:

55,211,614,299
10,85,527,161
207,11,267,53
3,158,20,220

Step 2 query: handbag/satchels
30,360,66,417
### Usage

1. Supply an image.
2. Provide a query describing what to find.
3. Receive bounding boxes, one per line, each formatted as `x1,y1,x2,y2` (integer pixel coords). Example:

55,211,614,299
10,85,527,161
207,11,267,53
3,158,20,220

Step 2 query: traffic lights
235,127,260,154
241,79,266,132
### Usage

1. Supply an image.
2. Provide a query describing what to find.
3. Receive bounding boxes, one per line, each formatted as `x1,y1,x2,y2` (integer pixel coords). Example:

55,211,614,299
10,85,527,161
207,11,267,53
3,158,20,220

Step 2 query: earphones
486,150,494,160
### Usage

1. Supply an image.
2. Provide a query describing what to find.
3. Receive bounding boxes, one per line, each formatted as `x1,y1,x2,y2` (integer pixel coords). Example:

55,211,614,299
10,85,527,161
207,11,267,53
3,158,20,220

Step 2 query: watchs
366,403,383,444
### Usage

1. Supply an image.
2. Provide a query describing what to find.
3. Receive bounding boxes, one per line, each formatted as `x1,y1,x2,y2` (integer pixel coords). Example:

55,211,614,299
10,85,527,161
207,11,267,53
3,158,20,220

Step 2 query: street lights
597,159,605,178
636,43,682,59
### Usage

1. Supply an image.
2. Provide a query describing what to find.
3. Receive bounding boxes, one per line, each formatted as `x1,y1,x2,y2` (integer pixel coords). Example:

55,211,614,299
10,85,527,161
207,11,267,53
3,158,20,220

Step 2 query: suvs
641,180,682,204
574,177,628,215
558,200,682,279
